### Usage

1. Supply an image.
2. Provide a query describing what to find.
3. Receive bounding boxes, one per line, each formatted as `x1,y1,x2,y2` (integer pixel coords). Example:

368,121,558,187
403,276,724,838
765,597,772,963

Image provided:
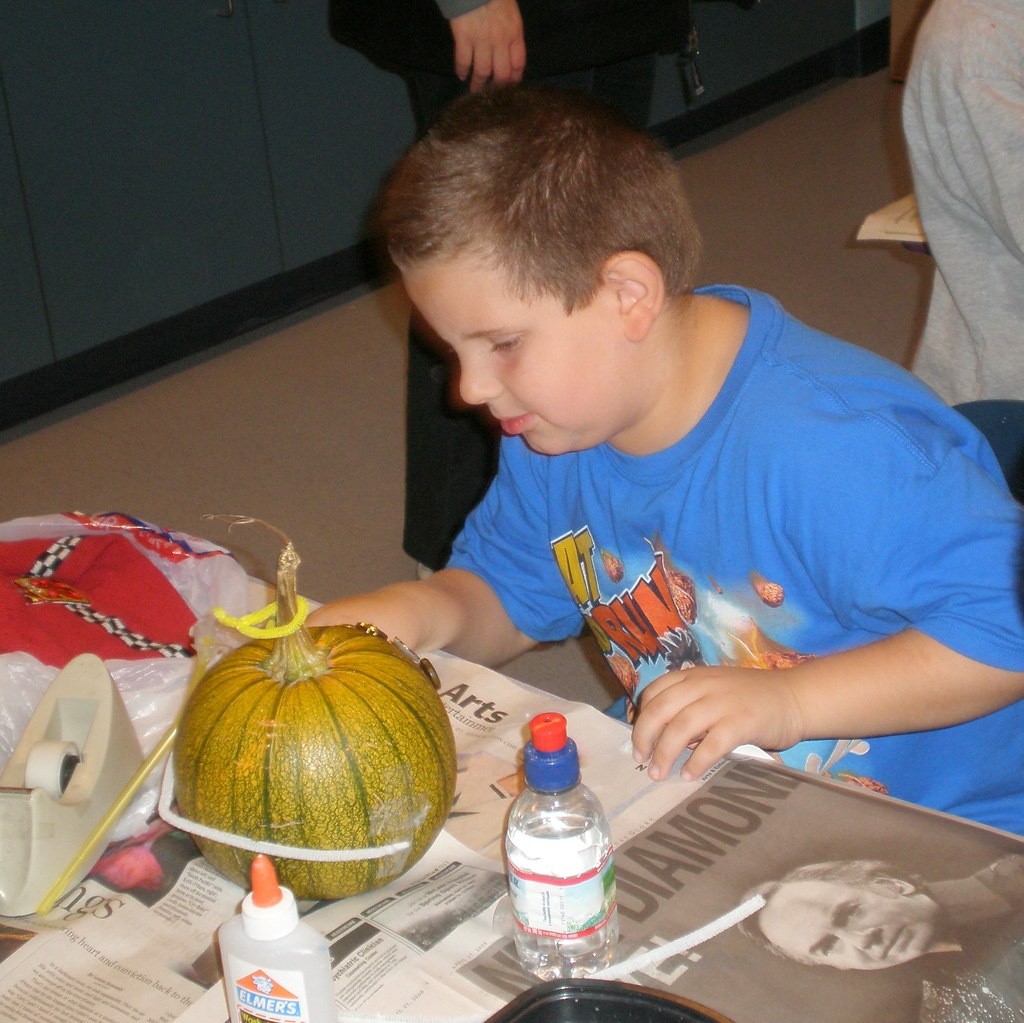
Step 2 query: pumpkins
174,541,456,900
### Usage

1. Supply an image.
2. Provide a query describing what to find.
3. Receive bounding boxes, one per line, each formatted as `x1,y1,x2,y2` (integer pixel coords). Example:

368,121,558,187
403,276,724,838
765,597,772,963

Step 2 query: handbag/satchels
0,510,247,842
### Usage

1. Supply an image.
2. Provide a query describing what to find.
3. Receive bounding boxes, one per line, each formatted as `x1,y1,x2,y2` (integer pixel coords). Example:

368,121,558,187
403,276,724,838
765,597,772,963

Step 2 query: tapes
24,740,82,794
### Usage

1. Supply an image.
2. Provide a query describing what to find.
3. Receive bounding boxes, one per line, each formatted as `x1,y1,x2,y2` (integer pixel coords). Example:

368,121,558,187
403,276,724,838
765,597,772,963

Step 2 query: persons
328,0,691,581
739,850,1024,1023
902,0,1024,506
301,89,1024,838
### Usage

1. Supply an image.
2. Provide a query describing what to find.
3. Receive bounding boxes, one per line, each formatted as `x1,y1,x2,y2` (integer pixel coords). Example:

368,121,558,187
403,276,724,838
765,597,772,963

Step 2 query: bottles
505,712,618,985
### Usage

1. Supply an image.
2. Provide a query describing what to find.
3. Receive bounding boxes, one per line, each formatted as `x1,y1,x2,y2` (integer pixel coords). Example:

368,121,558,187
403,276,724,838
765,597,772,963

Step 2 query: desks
0,593,1024,1023
